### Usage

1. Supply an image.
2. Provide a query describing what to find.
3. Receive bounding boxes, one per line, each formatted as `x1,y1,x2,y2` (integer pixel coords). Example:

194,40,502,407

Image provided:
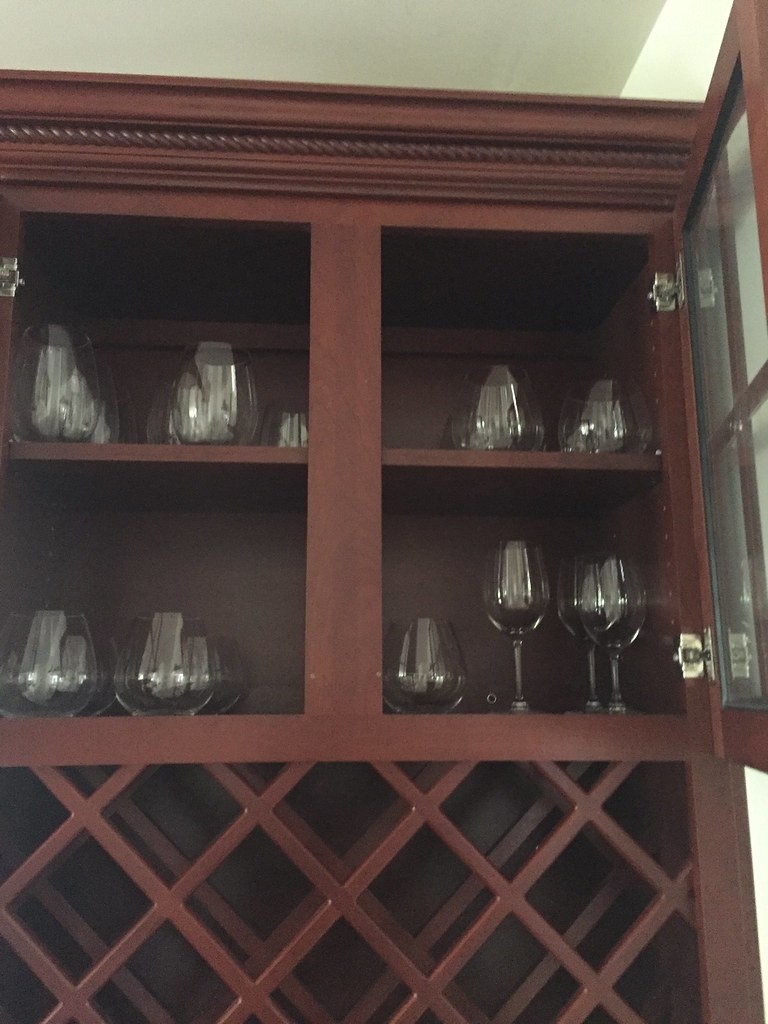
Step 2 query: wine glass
575,554,648,716
557,556,620,715
484,539,550,714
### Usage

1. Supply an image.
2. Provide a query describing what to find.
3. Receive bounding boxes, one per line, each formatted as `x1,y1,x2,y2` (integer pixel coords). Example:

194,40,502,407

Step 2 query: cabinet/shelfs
0,0,768,1024
